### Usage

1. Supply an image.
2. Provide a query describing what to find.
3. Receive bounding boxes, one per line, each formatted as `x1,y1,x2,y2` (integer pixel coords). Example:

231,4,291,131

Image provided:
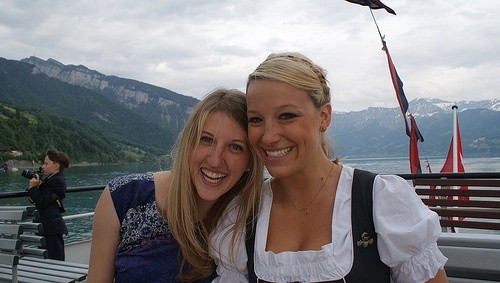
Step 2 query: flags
344,0,471,233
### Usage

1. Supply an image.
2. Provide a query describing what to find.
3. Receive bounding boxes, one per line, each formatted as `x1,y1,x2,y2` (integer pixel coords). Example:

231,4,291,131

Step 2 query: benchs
396,172,500,283
0,205,88,283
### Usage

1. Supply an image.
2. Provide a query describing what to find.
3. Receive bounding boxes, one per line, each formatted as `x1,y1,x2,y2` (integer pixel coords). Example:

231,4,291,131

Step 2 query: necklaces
283,162,334,212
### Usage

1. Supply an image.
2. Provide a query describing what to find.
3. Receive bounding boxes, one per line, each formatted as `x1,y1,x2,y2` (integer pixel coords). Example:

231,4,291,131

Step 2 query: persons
27,148,70,261
208,52,450,283
87,89,266,283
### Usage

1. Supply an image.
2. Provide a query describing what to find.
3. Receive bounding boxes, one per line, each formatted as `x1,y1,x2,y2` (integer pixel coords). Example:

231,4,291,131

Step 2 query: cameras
21,164,44,180
